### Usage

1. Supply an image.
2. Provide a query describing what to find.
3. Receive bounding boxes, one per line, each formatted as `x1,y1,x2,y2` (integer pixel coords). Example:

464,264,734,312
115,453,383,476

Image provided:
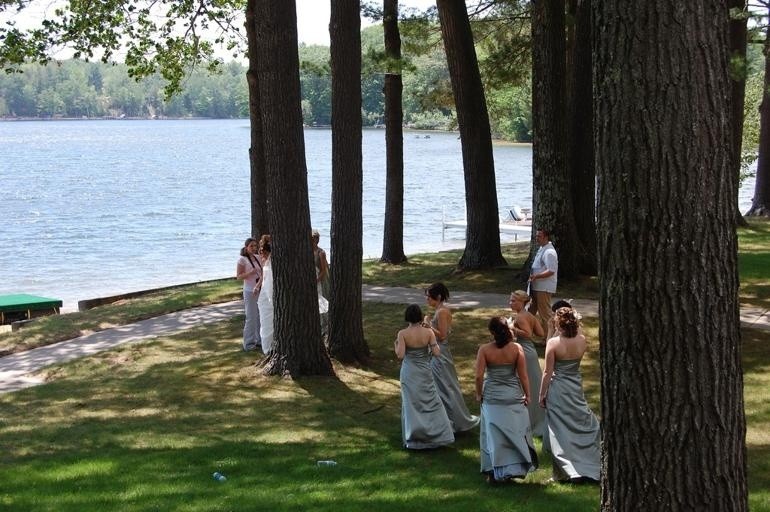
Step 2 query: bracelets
255,287,260,292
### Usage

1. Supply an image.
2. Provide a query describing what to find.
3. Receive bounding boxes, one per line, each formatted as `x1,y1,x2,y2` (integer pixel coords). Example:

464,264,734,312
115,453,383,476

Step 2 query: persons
249,233,276,356
540,300,574,457
505,288,547,439
236,236,265,353
473,314,540,487
392,302,455,452
421,280,482,438
537,305,603,487
526,226,560,349
309,228,332,340
